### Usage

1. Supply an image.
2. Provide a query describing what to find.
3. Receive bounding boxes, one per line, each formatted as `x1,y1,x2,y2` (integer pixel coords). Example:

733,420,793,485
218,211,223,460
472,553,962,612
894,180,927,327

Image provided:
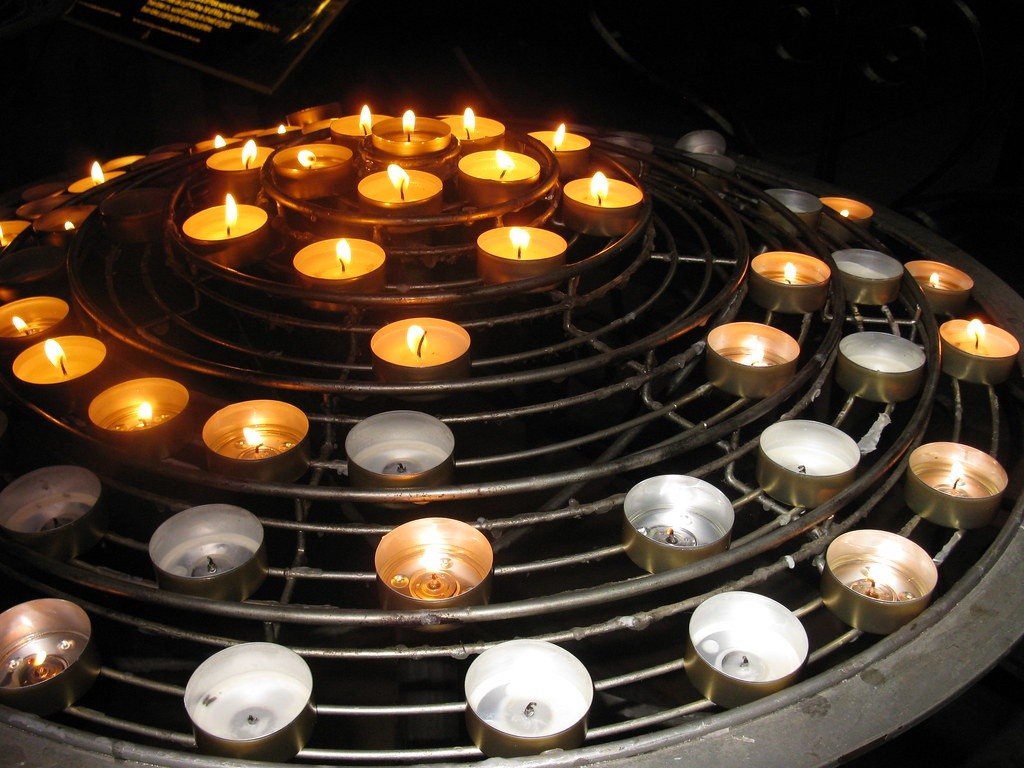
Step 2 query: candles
371,110,452,156
330,104,394,145
272,143,353,202
189,134,243,169
0,154,145,252
205,138,275,201
293,237,387,311
342,317,1019,759
357,164,443,217
256,125,302,147
476,224,568,292
749,189,975,314
563,171,643,238
182,194,270,267
1,333,311,768
441,107,506,153
527,122,591,180
457,148,541,209
